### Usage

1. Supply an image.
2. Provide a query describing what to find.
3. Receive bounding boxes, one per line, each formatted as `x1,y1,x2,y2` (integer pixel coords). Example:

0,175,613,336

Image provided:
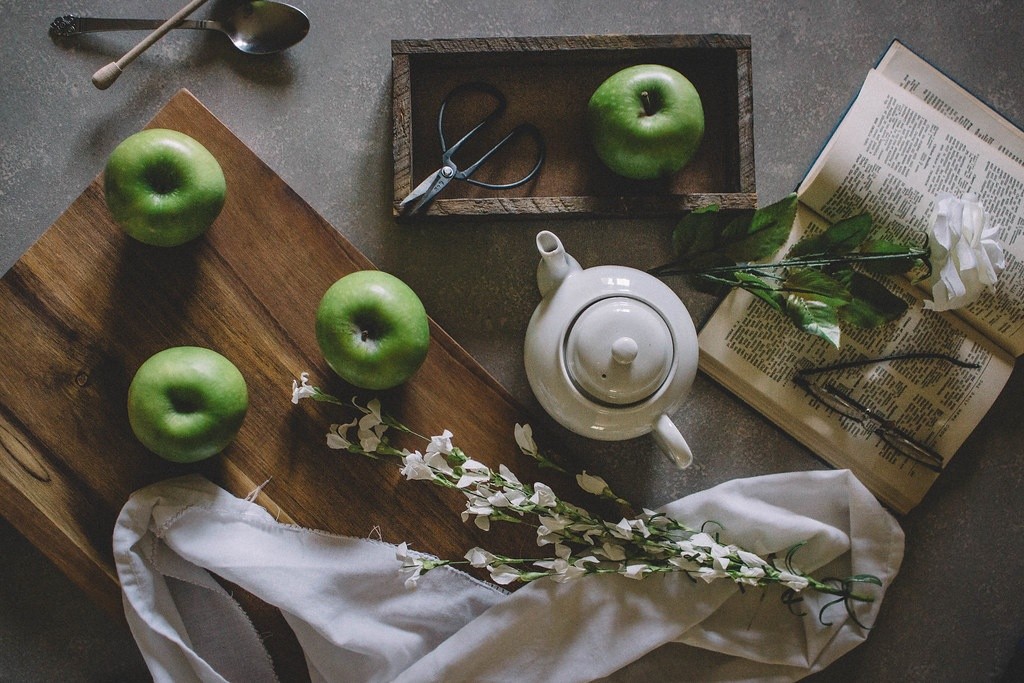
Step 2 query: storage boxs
391,33,758,221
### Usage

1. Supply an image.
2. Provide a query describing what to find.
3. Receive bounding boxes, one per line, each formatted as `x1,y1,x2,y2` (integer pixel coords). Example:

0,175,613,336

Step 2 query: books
698,40,1024,514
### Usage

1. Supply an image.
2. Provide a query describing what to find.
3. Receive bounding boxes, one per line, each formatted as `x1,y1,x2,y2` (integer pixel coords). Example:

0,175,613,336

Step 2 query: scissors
398,85,545,218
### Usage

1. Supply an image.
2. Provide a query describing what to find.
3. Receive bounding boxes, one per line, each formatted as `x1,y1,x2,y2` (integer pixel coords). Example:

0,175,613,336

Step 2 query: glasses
791,352,983,477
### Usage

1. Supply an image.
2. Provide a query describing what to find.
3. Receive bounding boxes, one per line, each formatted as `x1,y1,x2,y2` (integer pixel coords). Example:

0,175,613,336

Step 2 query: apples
105,128,227,248
314,270,429,390
587,63,706,180
128,346,249,463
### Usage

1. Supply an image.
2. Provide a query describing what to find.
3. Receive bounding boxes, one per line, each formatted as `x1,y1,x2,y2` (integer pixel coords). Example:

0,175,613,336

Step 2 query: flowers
291,371,885,634
646,188,1015,347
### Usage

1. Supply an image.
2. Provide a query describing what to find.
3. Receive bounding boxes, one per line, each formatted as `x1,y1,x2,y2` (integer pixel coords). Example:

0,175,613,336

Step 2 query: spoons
49,0,310,55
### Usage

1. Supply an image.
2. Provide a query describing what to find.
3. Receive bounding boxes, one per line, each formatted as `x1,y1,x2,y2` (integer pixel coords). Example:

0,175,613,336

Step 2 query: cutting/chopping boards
1,88,623,683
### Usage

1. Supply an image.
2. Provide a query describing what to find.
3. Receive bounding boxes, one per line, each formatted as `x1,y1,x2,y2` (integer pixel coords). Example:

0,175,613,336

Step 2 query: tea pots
524,230,698,468
